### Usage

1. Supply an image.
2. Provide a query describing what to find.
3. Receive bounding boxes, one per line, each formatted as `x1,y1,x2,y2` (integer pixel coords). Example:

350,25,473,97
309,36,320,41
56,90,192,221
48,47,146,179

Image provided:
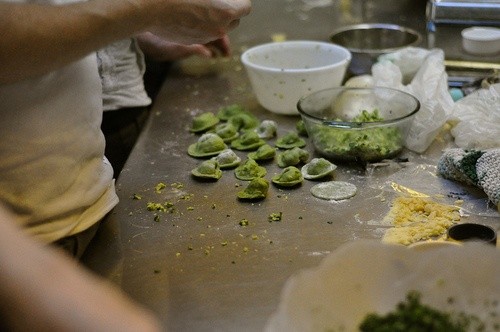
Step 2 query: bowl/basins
461,27,500,56
327,21,421,77
297,85,421,170
241,40,352,115
265,239,500,331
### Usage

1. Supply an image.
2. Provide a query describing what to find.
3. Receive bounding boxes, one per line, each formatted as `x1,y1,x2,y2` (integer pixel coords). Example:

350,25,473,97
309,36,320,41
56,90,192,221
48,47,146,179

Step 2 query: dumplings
188,104,337,200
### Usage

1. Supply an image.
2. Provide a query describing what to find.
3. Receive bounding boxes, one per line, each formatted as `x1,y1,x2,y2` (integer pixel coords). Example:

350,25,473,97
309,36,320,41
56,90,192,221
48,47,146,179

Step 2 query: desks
77,0,500,332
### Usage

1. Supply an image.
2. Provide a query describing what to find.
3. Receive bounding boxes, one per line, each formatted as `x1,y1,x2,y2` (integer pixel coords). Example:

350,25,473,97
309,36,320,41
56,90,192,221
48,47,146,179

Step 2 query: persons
0,0,253,263
93,31,234,186
0,202,169,332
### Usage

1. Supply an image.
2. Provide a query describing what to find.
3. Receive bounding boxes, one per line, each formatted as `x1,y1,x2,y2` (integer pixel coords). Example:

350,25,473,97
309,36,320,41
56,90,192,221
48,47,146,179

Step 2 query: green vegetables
357,289,500,332
311,109,403,161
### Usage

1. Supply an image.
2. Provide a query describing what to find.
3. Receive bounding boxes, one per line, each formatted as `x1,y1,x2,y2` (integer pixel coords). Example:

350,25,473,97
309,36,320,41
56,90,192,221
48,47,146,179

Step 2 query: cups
447,222,497,247
